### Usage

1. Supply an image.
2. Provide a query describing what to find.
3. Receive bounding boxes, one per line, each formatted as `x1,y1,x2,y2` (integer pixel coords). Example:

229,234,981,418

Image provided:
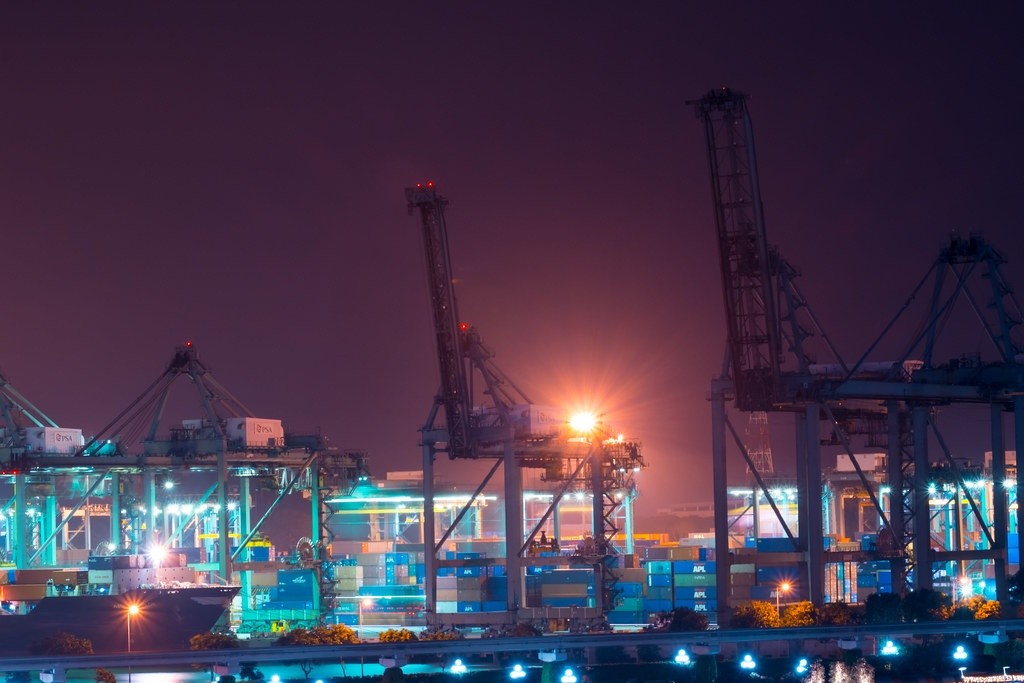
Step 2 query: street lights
127,604,139,682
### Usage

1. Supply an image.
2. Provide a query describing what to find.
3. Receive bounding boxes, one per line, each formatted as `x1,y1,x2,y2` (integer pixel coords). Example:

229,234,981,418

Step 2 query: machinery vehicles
403,181,563,460
685,84,822,413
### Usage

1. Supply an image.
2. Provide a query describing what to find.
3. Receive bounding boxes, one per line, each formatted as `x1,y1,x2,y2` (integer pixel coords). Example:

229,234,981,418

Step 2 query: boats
0,586,242,658
